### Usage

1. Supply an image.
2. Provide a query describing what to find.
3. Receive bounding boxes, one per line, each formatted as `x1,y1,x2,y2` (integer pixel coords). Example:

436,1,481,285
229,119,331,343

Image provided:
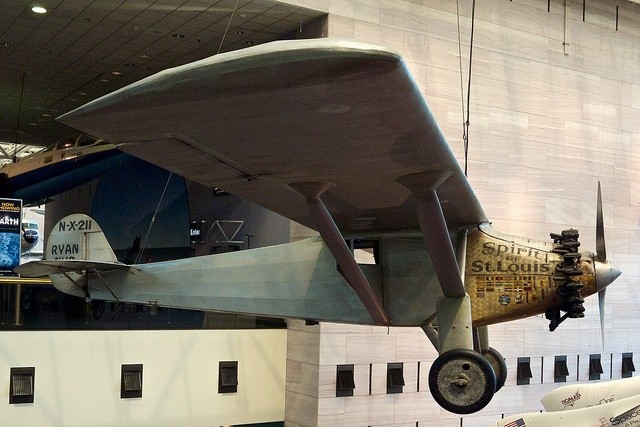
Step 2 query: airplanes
21,224,38,254
13,37,622,414
0,133,132,207
